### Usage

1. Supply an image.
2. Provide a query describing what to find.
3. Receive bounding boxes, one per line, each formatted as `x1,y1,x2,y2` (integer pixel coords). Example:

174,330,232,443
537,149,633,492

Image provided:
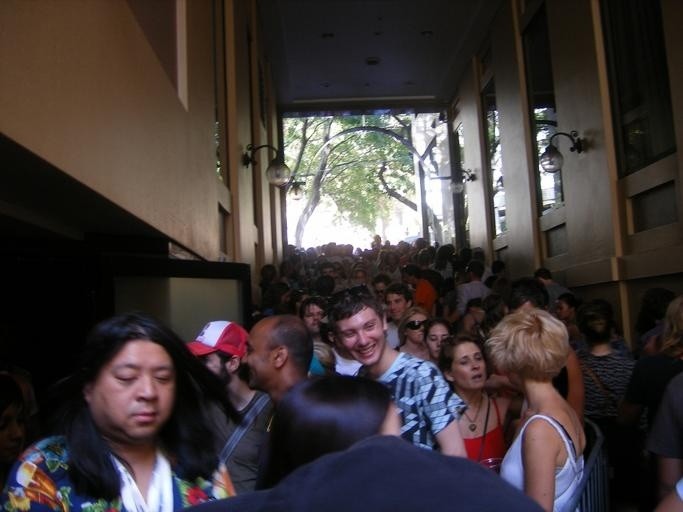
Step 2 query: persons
1,232,683,511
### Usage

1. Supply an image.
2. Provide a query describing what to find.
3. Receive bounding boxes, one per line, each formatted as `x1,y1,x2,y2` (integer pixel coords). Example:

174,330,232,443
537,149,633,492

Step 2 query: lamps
540,129,588,172
280,175,306,200
448,167,475,194
242,142,290,187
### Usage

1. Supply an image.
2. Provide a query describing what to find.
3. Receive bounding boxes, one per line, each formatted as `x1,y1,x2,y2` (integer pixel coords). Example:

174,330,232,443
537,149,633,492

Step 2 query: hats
185,319,250,356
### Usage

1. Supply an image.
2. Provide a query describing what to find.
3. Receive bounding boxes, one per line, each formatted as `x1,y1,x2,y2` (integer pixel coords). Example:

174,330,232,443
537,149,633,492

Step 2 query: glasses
406,321,429,330
